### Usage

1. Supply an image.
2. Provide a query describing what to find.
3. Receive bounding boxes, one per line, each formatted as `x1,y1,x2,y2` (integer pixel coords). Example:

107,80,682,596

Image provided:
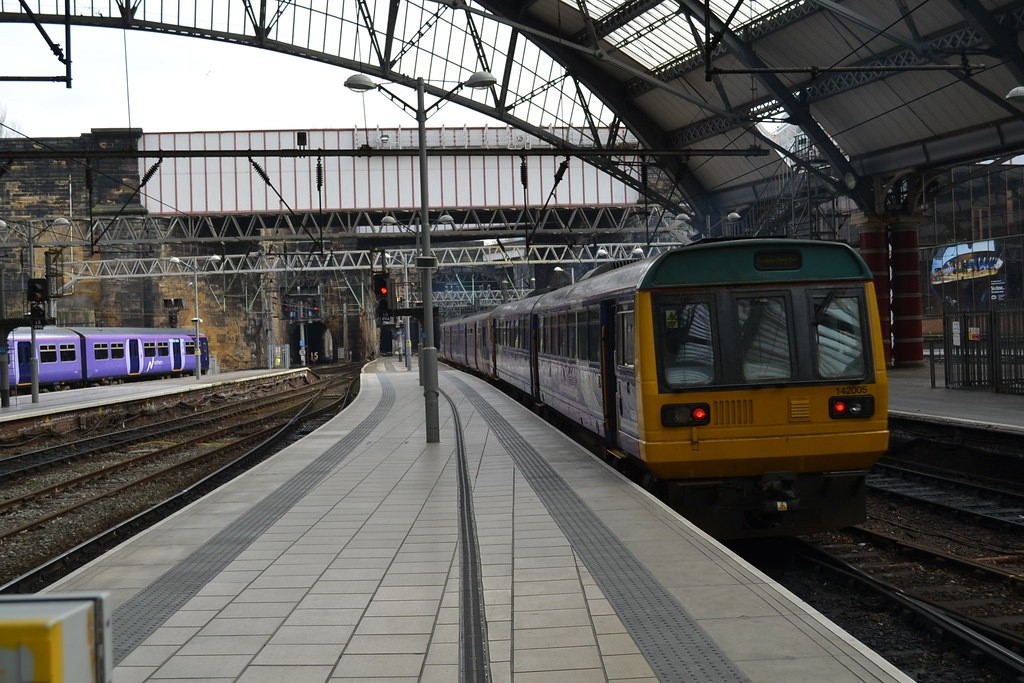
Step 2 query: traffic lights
282,304,289,315
379,280,388,297
29,278,47,302
313,298,319,314
31,303,45,329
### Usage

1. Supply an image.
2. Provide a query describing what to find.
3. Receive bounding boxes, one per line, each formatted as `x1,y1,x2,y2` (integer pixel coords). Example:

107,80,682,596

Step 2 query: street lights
343,71,498,443
381,214,453,386
385,252,412,372
170,254,221,378
553,265,574,284
675,211,741,237
0,217,70,403
597,247,643,260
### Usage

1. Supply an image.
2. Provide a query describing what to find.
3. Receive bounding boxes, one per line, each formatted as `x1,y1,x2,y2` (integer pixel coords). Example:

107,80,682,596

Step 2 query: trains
438,236,890,528
7,327,209,393
